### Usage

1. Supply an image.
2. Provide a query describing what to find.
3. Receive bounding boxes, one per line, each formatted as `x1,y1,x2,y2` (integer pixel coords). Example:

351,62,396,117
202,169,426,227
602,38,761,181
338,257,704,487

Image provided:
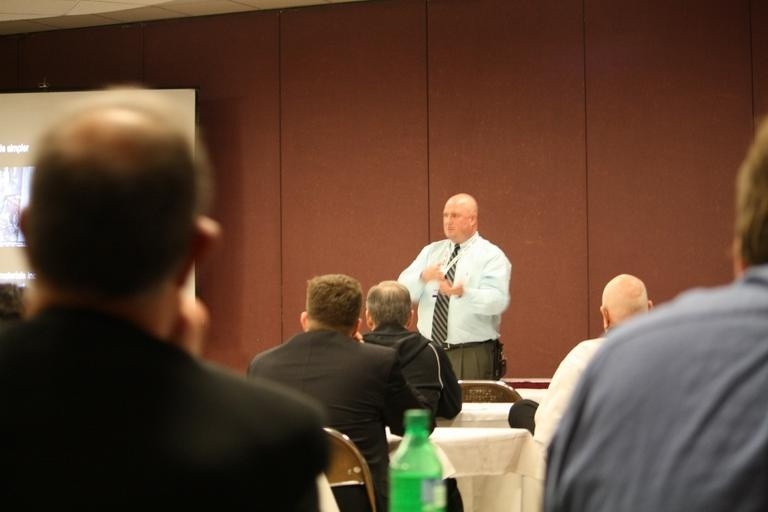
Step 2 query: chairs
458,379,523,402
323,427,377,512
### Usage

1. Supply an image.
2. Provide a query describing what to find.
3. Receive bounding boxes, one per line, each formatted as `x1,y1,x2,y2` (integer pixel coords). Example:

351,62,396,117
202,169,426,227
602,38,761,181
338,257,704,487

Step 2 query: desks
384,427,546,512
435,403,514,427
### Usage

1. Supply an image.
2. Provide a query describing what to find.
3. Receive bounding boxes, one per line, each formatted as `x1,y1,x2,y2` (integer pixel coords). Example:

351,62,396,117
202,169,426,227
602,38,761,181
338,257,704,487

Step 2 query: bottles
386,408,448,512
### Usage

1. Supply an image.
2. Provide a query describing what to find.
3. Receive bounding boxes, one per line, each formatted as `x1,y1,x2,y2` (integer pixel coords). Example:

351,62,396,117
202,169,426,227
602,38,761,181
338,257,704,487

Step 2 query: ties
432,243,459,349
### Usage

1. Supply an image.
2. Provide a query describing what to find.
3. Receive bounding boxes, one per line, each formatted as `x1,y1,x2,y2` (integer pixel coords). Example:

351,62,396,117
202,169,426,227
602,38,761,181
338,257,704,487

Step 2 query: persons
0,94,327,510
536,113,768,510
508,272,656,449
360,279,464,440
245,273,468,511
394,192,514,381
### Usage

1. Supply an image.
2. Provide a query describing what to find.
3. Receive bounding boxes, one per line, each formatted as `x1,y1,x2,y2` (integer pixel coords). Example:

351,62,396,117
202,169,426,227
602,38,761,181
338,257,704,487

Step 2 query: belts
434,340,498,352
